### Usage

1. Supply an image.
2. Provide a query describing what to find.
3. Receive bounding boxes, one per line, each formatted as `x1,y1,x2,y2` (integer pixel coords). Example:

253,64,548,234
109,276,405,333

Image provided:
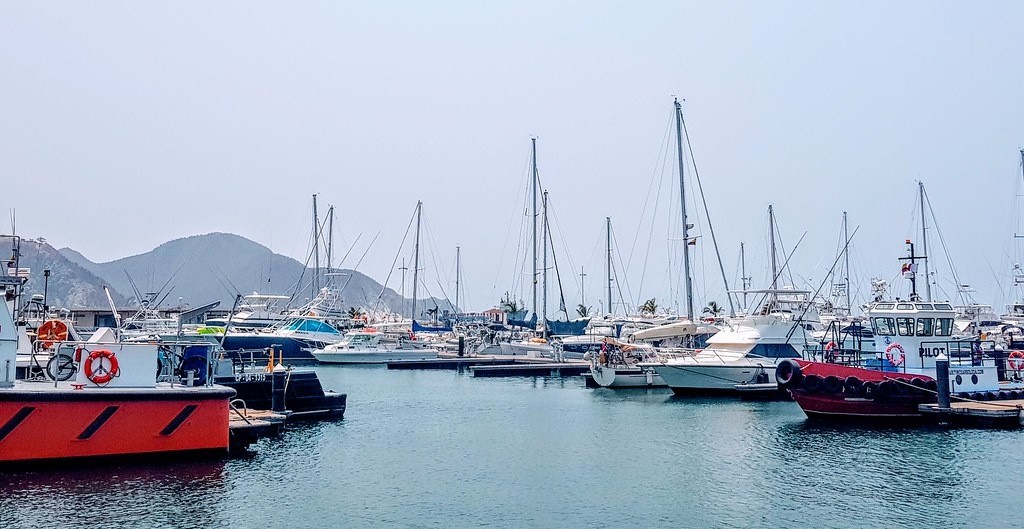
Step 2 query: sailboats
210,99,1022,425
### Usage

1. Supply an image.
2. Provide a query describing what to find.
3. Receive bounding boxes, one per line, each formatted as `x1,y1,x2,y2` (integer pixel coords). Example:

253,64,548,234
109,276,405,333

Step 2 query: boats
1,206,346,461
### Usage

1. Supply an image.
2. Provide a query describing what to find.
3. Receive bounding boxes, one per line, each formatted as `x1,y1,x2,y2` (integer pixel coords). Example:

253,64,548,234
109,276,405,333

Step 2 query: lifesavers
798,373,936,400
1009,349,1024,369
886,344,905,366
960,389,1023,401
824,340,838,362
775,360,801,387
38,321,68,348
84,350,117,383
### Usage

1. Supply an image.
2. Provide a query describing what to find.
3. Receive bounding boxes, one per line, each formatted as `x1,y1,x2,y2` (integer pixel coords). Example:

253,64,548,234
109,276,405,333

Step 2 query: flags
688,239,696,245
902,263,917,275
